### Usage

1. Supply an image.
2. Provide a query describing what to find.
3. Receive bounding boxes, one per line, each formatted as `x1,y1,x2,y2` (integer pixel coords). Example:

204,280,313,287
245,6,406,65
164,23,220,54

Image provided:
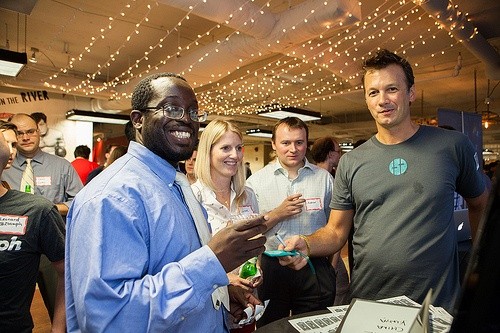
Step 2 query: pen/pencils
274,233,287,247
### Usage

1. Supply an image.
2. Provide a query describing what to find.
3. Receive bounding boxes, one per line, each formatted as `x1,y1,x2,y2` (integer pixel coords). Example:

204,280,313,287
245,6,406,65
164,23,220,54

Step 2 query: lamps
65,45,138,123
258,77,324,121
246,125,273,139
477,80,499,129
29,46,39,63
0,11,29,78
341,103,362,152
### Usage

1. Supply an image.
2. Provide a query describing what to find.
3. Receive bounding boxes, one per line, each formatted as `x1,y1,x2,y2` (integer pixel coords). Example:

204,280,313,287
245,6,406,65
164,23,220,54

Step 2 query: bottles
25,185,31,193
238,255,258,282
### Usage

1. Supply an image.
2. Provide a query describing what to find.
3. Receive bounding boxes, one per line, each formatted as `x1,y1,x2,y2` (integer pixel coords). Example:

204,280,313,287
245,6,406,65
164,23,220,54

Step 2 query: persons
278,49,488,315
0,113,127,333
185,118,264,333
65,72,268,333
246,117,367,328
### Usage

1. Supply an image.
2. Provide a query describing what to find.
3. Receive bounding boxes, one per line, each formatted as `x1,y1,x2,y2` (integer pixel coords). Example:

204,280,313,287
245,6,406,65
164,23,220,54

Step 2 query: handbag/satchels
337,288,432,333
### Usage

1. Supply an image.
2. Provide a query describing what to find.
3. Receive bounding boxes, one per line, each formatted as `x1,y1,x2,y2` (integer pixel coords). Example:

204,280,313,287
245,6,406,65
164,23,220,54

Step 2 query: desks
250,307,343,333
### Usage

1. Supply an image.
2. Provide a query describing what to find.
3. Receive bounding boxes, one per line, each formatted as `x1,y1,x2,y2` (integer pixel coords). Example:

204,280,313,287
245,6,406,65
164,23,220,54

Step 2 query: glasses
17,129,38,138
135,104,209,122
332,149,342,155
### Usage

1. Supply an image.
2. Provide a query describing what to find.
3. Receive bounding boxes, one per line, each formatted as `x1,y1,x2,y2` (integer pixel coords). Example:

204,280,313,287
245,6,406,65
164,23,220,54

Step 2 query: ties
175,170,230,311
20,158,35,194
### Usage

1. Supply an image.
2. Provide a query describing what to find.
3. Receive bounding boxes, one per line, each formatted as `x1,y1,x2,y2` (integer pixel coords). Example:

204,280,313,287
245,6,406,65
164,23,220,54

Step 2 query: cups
285,188,306,216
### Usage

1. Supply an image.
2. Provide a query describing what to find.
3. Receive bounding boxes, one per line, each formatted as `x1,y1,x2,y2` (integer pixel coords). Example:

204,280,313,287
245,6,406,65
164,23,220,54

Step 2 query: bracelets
300,234,312,256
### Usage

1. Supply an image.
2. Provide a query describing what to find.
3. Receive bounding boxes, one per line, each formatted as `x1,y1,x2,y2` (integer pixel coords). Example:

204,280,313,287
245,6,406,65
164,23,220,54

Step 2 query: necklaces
220,192,230,207
